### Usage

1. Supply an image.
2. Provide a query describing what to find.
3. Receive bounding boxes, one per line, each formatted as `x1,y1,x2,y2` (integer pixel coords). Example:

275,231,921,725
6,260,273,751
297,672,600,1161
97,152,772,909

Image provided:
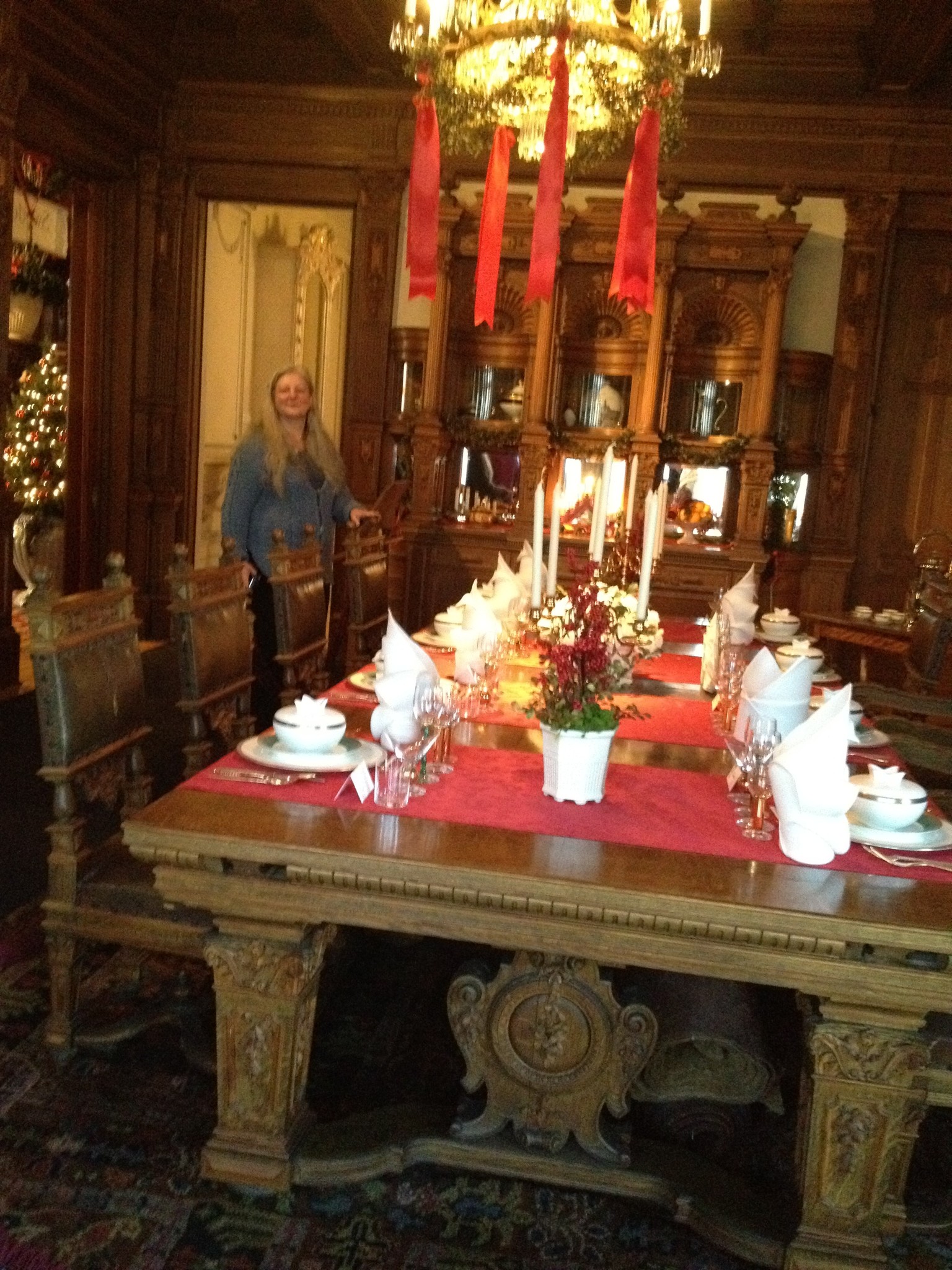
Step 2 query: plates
844,808,952,853
237,734,390,773
811,667,841,684
847,726,892,750
347,668,397,693
752,631,817,644
411,629,463,651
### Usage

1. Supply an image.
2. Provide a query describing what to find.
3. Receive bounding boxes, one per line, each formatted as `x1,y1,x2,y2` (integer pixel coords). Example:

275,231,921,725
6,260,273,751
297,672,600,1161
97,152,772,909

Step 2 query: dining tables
121,611,952,1269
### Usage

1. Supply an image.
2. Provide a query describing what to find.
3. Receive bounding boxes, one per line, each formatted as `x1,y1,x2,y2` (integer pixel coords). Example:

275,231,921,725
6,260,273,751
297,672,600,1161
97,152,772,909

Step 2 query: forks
860,842,952,871
206,774,317,786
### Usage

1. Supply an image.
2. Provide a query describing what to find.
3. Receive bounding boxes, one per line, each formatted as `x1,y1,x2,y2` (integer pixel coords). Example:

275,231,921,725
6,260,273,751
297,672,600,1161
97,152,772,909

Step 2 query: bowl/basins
433,612,463,637
759,612,800,635
272,705,345,752
847,773,927,830
807,696,862,730
775,644,824,674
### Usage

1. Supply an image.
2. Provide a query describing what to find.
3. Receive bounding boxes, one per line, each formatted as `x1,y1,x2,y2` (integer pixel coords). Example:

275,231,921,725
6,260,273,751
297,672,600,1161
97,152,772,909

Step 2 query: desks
800,610,914,689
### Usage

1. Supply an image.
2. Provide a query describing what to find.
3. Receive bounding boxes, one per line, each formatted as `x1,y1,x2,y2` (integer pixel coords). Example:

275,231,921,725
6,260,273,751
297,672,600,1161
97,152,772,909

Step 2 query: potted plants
513,545,651,807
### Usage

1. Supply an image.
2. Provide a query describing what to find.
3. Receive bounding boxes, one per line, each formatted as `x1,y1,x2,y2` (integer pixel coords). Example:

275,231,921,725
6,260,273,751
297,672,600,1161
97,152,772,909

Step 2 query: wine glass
706,584,785,839
408,591,549,797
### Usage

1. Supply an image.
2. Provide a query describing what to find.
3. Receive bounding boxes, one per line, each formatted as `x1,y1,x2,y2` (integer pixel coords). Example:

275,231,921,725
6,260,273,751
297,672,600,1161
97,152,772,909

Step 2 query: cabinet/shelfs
377,170,813,638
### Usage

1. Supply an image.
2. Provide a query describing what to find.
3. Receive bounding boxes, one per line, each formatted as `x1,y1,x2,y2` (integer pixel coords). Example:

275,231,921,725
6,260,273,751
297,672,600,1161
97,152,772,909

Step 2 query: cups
374,758,411,808
853,604,905,625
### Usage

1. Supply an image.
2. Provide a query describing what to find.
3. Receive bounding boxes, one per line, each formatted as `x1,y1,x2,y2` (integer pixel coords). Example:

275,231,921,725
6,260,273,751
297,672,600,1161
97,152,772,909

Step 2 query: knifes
213,766,326,783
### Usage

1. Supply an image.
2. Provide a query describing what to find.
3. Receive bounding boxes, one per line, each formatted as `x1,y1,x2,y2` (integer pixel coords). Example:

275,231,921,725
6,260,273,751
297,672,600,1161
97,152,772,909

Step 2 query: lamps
389,1,722,177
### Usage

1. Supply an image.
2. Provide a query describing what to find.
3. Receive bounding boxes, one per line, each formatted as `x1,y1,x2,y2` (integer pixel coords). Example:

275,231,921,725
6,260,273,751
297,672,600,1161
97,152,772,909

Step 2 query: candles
530,442,669,621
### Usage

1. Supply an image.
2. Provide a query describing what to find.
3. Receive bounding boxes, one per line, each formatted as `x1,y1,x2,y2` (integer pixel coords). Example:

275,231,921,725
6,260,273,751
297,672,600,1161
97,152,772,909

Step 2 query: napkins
372,539,541,756
700,563,853,864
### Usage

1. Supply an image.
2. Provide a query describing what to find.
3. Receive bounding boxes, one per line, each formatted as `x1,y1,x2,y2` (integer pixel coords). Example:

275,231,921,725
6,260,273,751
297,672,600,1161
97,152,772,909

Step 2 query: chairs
264,525,330,713
851,677,952,1114
340,508,394,677
30,552,216,1089
166,540,256,786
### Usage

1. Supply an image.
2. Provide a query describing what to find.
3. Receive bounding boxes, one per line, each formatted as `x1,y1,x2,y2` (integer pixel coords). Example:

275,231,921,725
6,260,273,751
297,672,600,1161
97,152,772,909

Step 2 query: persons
466,446,518,508
219,364,384,737
